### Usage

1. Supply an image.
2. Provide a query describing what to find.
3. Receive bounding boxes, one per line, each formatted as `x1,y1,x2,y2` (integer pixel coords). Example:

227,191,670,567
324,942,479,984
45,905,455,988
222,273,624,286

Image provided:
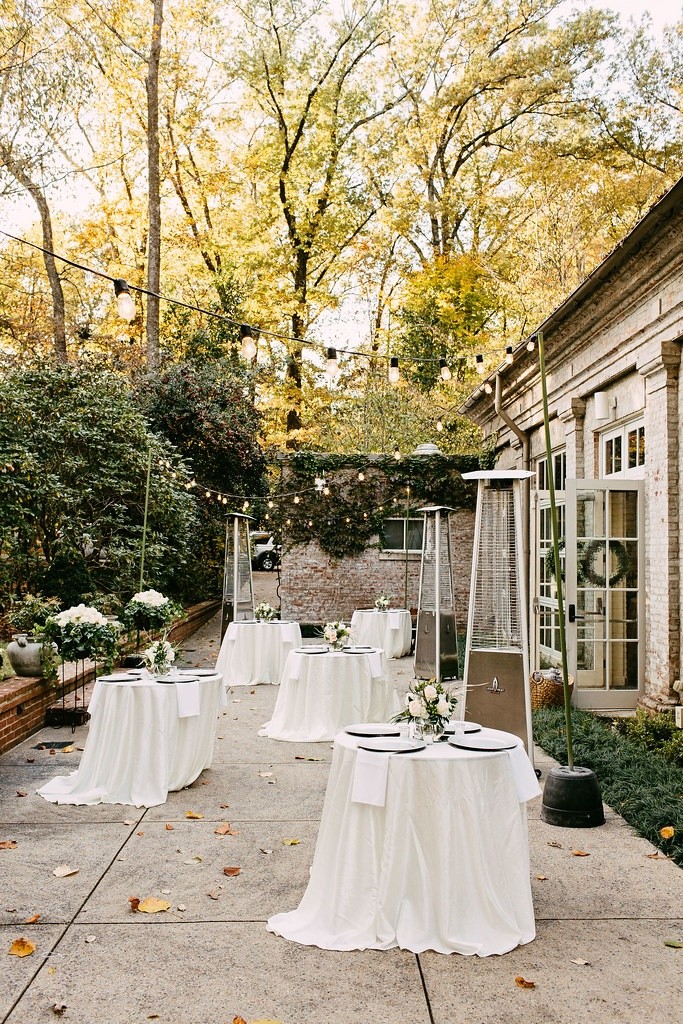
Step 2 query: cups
351,643,355,651
273,618,278,621
253,618,257,623
126,668,147,674
261,618,264,623
168,666,177,679
424,730,434,744
399,725,410,745
96,673,138,682
454,723,466,740
141,668,150,680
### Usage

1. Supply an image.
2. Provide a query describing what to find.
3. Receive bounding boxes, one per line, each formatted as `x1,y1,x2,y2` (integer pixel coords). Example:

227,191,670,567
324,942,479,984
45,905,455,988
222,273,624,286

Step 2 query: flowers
313,618,353,648
374,595,395,609
137,623,183,673
391,662,459,739
39,600,121,686
254,602,282,620
125,588,186,628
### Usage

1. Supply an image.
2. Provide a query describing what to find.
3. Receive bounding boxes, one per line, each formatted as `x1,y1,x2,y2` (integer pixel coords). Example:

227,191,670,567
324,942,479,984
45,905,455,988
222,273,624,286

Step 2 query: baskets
529,668,575,711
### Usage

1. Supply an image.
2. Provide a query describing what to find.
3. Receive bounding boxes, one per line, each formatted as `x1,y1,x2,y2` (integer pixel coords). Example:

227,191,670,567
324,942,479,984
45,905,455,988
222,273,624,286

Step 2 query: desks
259,647,405,743
36,670,227,808
216,620,303,687
267,721,536,958
348,607,413,659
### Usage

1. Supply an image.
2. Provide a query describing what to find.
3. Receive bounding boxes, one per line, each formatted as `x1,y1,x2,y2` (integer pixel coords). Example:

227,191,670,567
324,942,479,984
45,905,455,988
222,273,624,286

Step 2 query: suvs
254,535,282,572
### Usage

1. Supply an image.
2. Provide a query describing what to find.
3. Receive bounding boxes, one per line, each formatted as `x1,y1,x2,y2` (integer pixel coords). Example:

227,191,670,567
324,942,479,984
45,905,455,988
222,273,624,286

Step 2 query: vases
379,607,388,612
135,613,152,630
56,635,91,659
8,632,59,676
421,720,435,744
155,664,168,675
260,617,269,623
330,638,342,651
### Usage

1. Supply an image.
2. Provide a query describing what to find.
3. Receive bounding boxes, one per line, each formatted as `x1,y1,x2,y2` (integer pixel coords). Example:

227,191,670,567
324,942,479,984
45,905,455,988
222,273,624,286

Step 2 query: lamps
595,391,616,420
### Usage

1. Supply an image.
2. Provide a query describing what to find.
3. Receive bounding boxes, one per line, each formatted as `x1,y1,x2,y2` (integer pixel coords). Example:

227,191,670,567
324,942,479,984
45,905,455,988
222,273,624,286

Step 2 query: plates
395,609,406,611
342,645,370,649
356,609,374,612
295,649,329,653
343,648,375,654
155,675,201,683
374,607,378,612
268,621,289,624
358,737,426,752
179,669,217,675
441,720,482,733
448,733,518,749
301,645,330,650
367,608,374,610
382,611,398,613
344,722,410,735
234,621,256,623
